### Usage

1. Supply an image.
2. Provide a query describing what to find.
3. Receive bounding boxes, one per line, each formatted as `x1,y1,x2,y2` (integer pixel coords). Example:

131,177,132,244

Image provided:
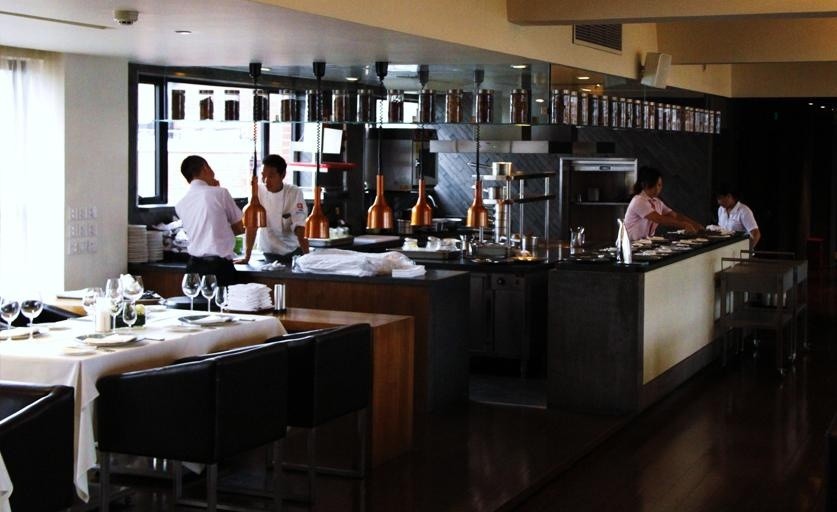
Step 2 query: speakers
638,52,673,90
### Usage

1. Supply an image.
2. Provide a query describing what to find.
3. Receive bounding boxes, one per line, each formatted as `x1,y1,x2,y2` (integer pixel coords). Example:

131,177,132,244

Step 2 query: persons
616,166,706,246
171,153,245,291
232,156,311,264
713,182,761,257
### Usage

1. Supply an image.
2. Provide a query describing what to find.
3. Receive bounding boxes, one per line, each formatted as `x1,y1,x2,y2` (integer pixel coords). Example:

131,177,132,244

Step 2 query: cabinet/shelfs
559,157,640,246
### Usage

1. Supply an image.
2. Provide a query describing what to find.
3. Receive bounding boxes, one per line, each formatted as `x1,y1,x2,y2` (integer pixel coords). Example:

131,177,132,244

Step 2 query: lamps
242,62,267,229
466,67,489,229
367,61,393,231
304,64,330,241
410,64,434,227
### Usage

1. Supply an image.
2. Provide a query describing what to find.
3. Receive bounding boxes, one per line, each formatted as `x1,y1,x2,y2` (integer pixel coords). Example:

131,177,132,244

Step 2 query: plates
127,223,165,264
598,229,731,262
1,326,37,340
75,335,136,345
178,315,233,325
226,305,273,312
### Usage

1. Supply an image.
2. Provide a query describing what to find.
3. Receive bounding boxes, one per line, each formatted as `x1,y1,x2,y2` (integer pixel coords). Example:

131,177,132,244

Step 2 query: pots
472,186,507,200
465,160,512,178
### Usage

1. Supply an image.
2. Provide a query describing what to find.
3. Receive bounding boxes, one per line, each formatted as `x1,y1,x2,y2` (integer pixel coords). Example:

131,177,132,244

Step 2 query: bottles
475,89,494,124
509,89,527,125
445,89,464,124
307,89,323,123
172,90,186,120
356,90,370,124
418,89,435,124
252,90,270,123
331,90,349,122
199,90,214,120
387,89,404,123
279,89,296,122
225,90,240,121
273,283,286,310
550,88,721,134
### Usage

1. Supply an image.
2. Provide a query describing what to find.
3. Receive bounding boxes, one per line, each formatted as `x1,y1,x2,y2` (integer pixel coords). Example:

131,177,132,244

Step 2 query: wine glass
180,273,201,314
0,298,20,340
201,275,216,315
214,286,228,312
19,298,43,340
81,275,144,333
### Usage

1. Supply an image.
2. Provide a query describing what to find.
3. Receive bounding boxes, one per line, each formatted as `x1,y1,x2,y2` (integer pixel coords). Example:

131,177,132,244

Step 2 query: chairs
1,383,74,512
1,302,82,329
